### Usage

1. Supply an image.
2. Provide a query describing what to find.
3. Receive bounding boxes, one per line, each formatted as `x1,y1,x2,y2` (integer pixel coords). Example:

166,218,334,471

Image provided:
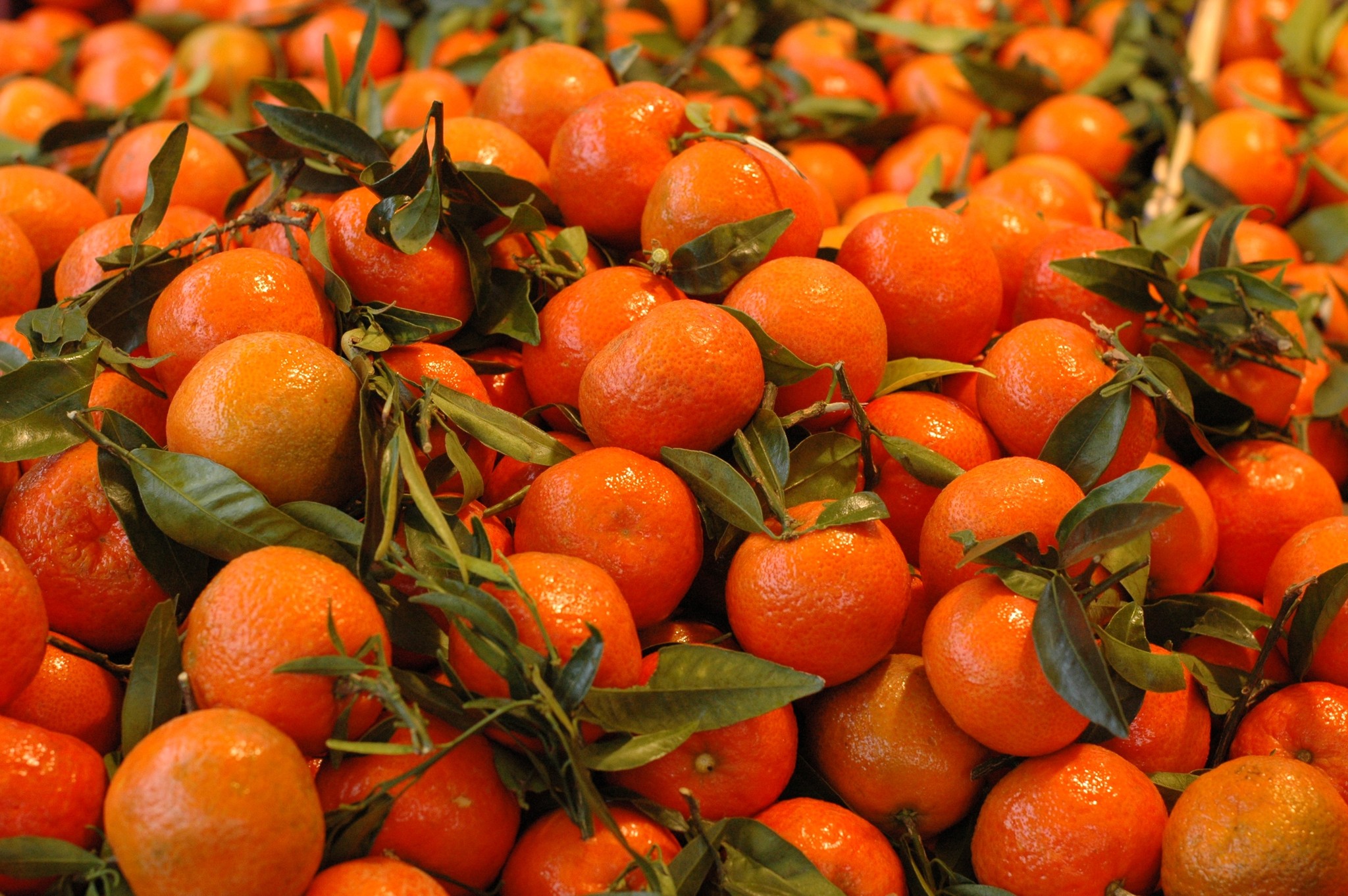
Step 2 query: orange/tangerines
0,1,1348,896
